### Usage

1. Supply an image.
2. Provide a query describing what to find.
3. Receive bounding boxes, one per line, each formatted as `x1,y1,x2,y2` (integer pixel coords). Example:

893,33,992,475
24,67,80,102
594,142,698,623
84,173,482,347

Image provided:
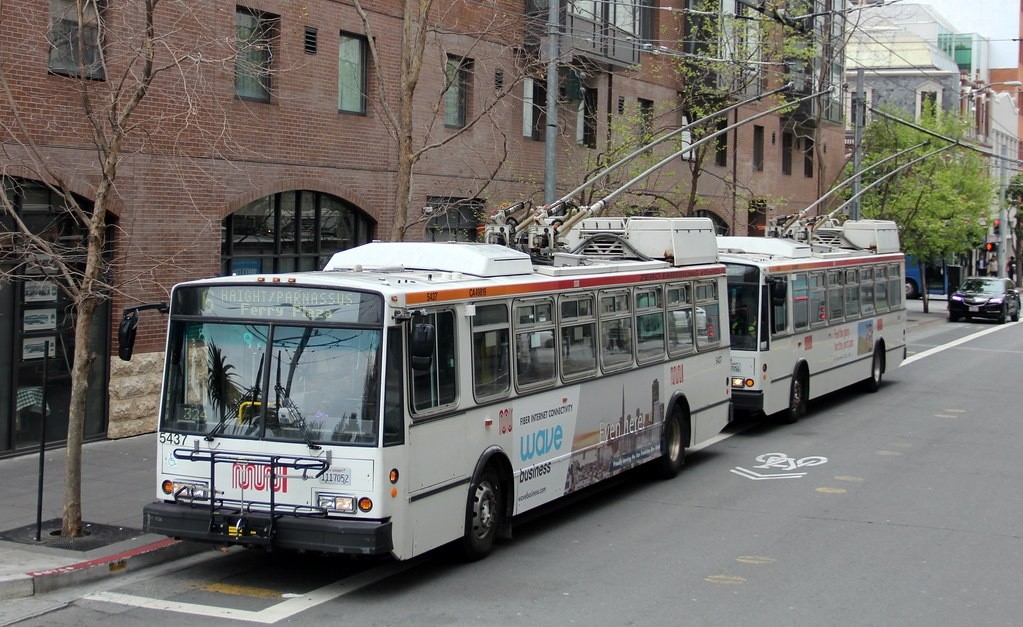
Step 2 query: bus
904,243,986,299
713,220,906,431
116,218,735,568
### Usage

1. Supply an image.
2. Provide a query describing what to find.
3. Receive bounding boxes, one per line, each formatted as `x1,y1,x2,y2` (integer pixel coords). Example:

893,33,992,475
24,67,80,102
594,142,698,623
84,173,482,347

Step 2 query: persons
976,254,998,277
1005,256,1016,280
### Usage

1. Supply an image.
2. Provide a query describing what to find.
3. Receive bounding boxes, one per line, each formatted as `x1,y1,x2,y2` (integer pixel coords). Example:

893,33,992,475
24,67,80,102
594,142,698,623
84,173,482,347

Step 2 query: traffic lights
984,242,995,251
994,219,1000,234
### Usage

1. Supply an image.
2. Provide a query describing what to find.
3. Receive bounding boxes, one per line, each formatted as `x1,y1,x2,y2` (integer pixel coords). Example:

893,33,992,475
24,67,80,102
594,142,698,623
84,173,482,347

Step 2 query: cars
948,275,1021,325
